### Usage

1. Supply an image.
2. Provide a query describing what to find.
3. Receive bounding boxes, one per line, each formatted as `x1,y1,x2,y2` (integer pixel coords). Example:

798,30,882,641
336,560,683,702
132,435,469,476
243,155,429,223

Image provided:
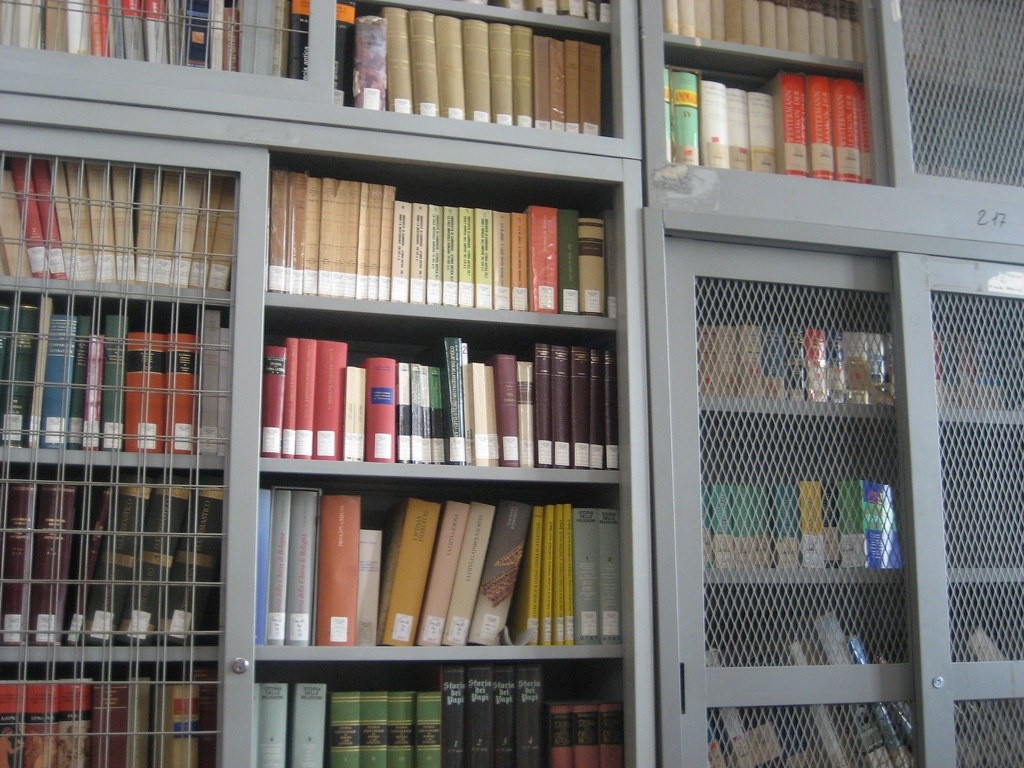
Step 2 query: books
1,475,624,768
1,151,607,315
696,322,1012,409
1,295,618,469
706,606,1024,768
663,0,877,187
1,0,613,137
702,478,903,570
